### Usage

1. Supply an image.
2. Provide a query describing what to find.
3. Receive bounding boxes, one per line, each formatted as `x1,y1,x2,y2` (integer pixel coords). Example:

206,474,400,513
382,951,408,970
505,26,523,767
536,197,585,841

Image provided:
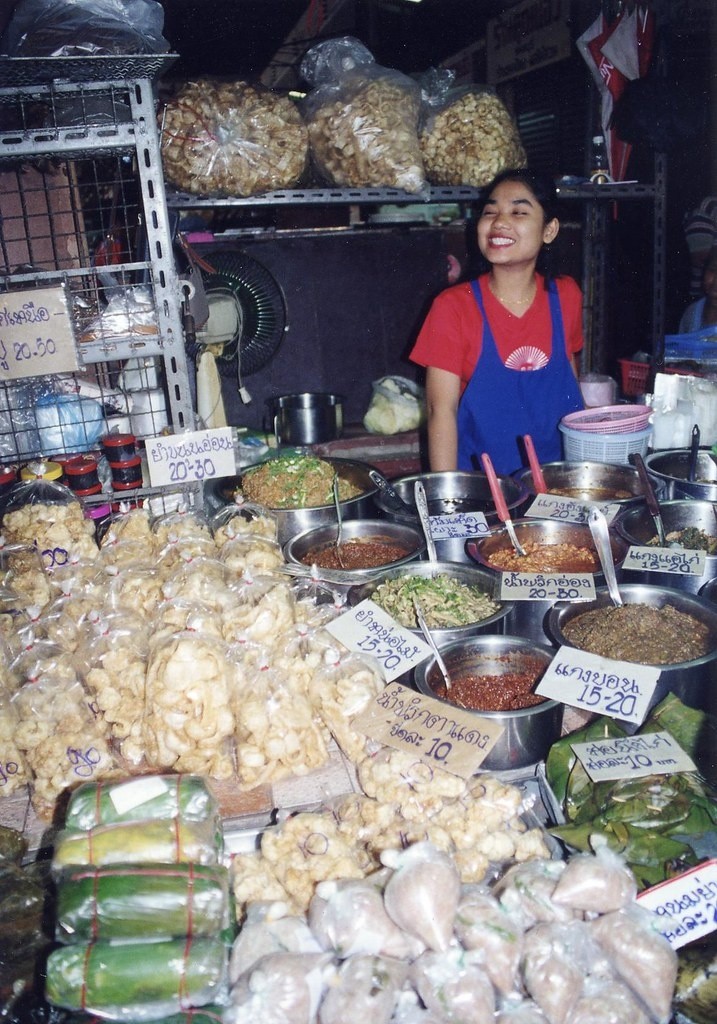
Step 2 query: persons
677,175,717,335
410,168,586,475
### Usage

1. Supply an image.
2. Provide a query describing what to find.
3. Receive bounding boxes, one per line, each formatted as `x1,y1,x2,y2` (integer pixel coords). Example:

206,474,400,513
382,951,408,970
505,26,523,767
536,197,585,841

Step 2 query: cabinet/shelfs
2,1,662,524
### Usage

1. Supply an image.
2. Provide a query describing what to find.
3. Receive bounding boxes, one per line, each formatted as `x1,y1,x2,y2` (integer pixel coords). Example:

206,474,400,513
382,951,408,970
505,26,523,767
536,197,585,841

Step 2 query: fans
182,249,288,456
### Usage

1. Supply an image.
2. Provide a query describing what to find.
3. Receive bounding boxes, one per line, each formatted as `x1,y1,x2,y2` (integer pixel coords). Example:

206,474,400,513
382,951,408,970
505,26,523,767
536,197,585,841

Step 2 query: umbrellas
577,0,656,218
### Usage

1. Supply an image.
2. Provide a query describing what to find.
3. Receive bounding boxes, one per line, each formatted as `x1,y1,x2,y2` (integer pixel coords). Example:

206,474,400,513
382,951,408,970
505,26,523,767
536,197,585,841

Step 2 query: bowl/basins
205,449,717,771
270,393,347,445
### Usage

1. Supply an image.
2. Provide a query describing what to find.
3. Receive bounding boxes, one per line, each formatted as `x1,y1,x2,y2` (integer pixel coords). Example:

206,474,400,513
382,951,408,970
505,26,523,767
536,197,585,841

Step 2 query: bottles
590,136,609,185
